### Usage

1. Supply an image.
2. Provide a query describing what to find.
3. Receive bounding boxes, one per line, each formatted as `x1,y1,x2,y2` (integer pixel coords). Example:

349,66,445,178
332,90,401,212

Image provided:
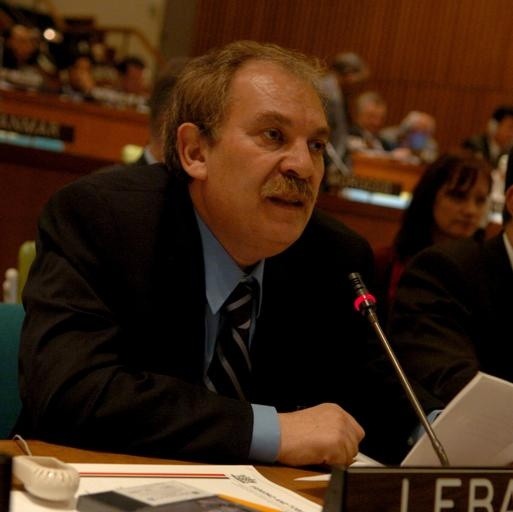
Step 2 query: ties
207,280,255,404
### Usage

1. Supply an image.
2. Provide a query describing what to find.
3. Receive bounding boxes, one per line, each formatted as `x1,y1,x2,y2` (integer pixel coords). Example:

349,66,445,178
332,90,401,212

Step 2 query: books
404,371,512,466
75,479,289,511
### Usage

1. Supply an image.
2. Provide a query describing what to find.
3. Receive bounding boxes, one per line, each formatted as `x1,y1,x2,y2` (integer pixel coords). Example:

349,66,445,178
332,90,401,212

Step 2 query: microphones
347,272,450,467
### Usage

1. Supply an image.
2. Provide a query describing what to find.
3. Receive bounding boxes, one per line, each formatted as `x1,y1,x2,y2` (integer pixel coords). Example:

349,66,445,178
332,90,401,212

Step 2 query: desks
0,82,513,512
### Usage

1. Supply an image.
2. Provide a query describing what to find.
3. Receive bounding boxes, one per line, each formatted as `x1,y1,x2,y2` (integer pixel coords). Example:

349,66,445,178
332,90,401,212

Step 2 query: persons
367,148,493,299
1,1,513,223
9,40,381,473
384,150,512,470
